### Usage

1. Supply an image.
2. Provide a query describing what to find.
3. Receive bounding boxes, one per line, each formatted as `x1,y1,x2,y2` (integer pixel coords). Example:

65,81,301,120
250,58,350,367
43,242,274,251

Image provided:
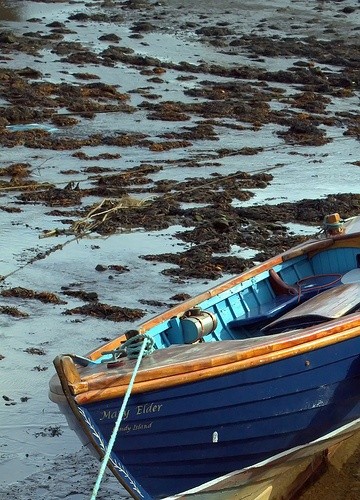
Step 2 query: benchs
227,272,339,337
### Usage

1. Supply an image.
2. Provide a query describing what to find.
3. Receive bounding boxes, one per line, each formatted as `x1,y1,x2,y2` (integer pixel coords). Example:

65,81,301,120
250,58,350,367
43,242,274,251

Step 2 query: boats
47,211,360,500
